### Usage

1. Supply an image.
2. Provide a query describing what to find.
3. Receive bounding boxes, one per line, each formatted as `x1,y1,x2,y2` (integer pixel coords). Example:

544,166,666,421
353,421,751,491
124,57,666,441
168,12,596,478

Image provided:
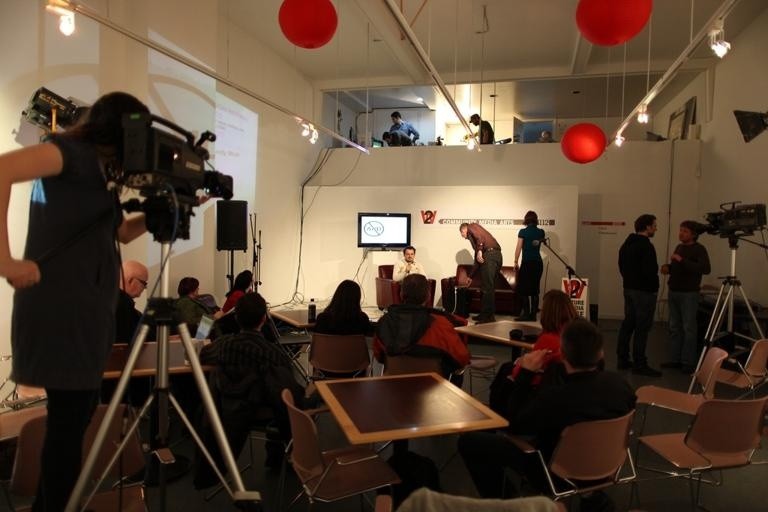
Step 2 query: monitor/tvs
358,212,410,251
371,139,383,148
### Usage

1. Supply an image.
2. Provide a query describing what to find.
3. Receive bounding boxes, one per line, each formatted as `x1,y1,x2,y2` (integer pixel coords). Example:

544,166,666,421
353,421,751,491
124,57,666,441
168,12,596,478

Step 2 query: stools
468,352,498,395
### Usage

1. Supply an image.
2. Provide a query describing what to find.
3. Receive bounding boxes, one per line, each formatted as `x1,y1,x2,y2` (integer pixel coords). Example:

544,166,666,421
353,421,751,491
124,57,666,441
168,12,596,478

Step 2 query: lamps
576,1,653,45
710,26,732,59
278,1,339,50
299,122,319,145
561,122,608,164
61,3,75,37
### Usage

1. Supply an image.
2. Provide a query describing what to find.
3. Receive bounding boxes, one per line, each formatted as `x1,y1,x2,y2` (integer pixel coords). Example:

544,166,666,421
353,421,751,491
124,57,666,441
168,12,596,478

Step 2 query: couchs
440,264,523,317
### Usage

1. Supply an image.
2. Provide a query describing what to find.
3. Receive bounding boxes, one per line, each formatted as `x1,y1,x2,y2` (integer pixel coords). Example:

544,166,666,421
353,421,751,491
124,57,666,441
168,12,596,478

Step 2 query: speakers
216,200,247,253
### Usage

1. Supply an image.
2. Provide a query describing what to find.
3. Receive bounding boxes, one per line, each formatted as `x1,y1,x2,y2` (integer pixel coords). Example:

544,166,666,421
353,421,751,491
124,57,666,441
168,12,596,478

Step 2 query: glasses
133,278,147,286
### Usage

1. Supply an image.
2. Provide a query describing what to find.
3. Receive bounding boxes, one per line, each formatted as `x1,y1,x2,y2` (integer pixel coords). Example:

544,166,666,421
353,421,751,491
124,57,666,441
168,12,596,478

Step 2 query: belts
484,248,500,251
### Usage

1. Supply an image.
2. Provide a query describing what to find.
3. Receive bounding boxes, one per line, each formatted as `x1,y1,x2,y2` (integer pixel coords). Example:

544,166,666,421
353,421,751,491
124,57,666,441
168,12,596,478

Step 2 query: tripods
8,193,247,512
687,238,766,394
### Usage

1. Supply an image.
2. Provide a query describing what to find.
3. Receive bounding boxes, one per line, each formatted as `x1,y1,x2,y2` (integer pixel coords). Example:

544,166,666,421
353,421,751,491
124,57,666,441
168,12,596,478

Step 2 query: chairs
281,389,402,511
495,407,637,512
635,346,729,486
626,395,768,512
266,311,314,385
1,377,177,512
380,355,469,377
690,339,767,466
300,333,375,380
375,264,436,311
397,486,566,512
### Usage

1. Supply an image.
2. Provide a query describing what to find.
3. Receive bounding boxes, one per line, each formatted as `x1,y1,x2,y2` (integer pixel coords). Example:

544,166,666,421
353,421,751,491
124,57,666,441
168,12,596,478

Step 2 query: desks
314,371,510,455
696,302,768,339
101,338,218,487
453,320,542,361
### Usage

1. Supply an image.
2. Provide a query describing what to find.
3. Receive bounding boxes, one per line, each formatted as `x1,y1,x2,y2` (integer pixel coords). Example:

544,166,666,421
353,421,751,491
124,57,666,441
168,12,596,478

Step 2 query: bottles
308,298,317,323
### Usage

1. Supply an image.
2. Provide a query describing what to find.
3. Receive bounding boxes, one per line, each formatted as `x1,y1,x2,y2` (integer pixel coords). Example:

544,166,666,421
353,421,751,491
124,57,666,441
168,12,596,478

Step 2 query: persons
514,212,545,321
469,114,494,144
0,91,213,512
212,270,306,476
661,220,711,374
390,111,420,146
383,130,412,146
618,213,663,378
113,263,210,419
490,290,635,511
537,131,557,143
392,246,428,280
459,223,503,326
308,275,469,390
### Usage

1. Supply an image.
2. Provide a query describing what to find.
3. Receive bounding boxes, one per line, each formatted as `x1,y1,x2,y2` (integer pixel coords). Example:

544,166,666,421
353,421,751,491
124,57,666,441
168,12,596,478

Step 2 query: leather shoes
472,314,496,324
514,314,537,321
619,364,661,377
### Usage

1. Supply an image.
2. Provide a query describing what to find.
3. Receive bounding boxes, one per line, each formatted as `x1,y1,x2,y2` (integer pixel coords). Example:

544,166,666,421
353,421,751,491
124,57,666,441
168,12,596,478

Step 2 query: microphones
532,238,547,246
